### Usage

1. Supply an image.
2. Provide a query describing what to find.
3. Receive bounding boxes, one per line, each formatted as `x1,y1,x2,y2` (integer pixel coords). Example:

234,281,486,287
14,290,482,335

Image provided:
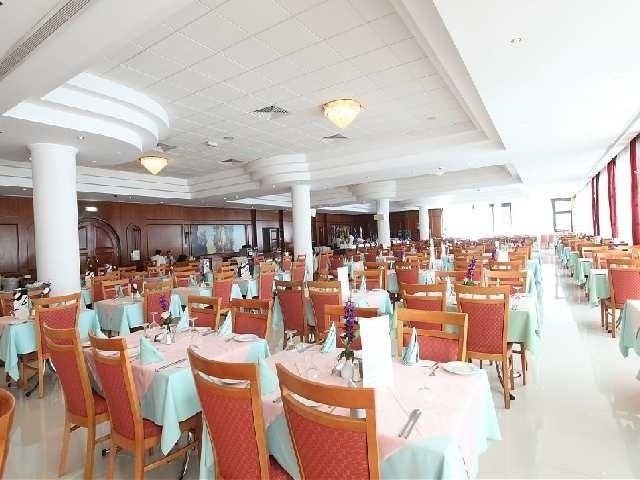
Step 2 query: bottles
352,362,360,382
130,292,133,299
166,333,171,345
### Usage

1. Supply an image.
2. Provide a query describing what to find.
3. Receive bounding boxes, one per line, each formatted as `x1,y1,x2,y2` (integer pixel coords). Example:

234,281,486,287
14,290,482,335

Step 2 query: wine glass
284,329,298,351
303,349,322,379
138,322,151,343
188,316,200,344
148,312,162,335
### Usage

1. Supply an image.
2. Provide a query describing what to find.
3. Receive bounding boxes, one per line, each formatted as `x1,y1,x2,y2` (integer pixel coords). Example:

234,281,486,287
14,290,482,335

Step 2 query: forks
428,366,439,377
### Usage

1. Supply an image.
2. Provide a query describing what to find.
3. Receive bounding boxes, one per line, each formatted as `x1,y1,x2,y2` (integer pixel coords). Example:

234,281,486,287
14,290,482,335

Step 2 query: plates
443,360,478,375
7,319,26,325
235,334,258,342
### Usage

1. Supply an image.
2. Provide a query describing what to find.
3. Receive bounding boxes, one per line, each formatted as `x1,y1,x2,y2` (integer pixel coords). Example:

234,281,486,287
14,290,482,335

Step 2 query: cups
402,347,407,358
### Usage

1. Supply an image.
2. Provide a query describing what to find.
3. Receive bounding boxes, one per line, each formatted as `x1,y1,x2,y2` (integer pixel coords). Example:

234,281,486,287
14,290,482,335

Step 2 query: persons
150,249,175,265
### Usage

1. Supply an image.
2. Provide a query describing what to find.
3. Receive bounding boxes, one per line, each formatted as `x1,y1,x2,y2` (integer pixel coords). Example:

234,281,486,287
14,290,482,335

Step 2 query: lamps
135,150,170,177
320,97,362,130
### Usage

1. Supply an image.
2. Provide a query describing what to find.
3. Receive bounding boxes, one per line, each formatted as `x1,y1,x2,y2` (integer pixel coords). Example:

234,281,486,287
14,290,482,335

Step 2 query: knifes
429,362,439,369
224,335,236,342
298,345,315,353
396,408,420,439
203,330,217,337
405,410,422,441
158,358,188,370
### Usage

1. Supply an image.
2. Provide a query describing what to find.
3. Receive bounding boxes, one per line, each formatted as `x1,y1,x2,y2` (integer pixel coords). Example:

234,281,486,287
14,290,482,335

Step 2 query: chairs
541,231,639,381
2,231,542,480
